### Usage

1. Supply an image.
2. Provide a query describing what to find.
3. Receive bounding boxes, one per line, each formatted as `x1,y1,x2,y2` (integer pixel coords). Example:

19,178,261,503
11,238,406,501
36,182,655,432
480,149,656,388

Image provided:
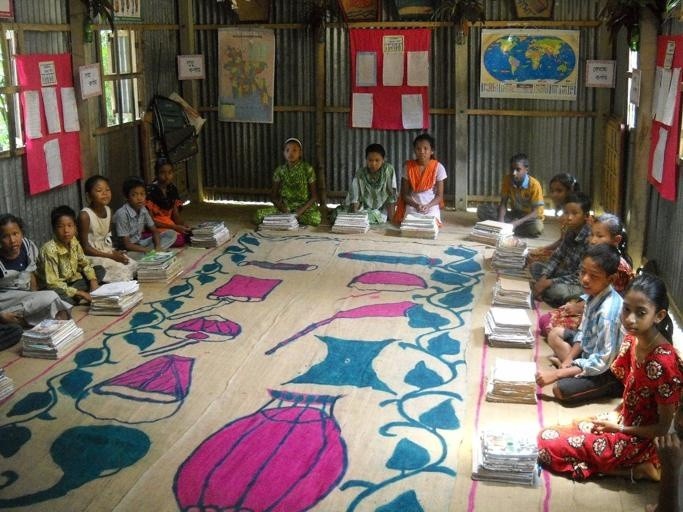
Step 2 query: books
0,369,19,401
463,219,536,349
256,214,300,236
484,356,538,405
399,212,439,240
470,430,539,489
189,220,232,248
331,211,370,234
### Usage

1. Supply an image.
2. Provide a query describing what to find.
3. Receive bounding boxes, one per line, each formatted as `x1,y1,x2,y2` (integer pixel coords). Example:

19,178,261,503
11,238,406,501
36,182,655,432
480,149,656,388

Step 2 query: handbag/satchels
151,94,198,165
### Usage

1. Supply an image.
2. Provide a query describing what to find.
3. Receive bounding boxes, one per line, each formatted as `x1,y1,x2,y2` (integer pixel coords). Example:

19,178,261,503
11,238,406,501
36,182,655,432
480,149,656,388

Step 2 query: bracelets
619,423,625,434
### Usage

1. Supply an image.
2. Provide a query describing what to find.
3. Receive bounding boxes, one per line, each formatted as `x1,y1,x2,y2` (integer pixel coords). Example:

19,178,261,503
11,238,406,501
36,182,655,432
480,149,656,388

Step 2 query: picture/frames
630,68,641,108
586,60,617,88
79,62,103,101
177,55,206,80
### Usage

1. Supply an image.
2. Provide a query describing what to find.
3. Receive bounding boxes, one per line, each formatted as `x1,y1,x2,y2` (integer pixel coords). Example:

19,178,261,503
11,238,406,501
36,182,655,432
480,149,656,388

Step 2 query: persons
326,144,398,224
588,213,637,291
521,173,580,271
530,190,593,308
251,136,322,225
537,275,683,482
392,133,447,228
536,243,626,405
1,156,193,360
477,153,546,238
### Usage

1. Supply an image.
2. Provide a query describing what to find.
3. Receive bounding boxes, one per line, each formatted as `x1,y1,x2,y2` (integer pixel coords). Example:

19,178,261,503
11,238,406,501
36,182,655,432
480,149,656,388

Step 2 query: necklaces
637,330,661,351
